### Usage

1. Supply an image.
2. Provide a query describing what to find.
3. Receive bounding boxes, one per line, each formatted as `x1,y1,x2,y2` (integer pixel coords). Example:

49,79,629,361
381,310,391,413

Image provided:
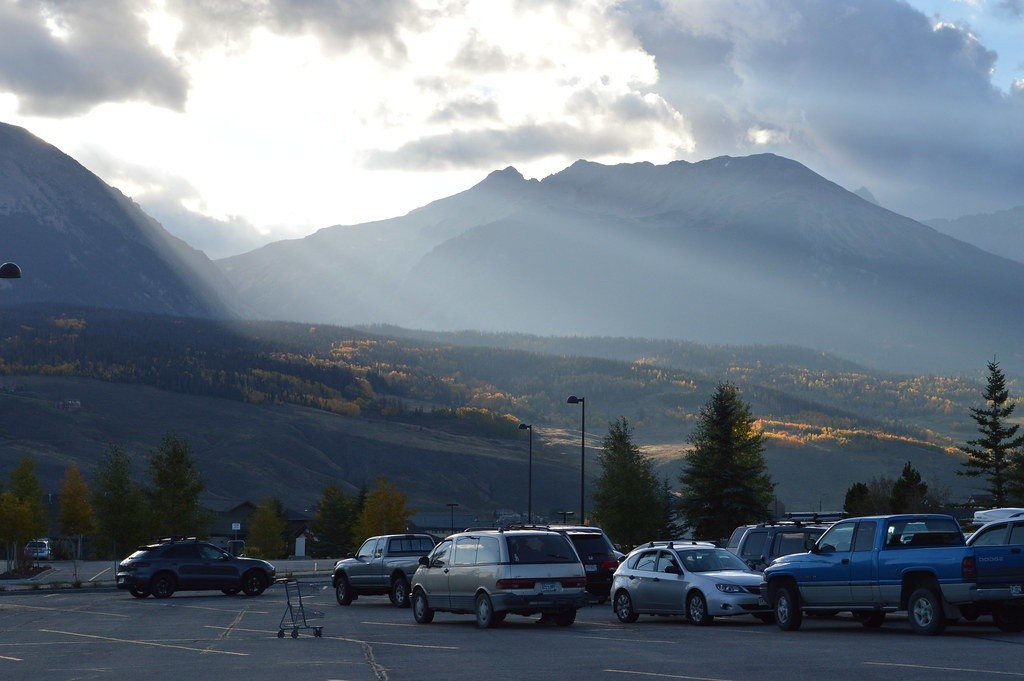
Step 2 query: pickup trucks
331,533,437,608
762,512,1024,637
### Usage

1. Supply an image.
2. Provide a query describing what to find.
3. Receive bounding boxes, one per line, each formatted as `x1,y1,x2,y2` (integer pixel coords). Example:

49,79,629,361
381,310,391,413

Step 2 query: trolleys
277,578,325,639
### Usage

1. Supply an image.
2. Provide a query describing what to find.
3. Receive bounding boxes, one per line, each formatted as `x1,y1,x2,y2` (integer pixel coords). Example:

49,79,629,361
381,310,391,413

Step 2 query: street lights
519,423,532,522
567,395,586,526
447,503,460,536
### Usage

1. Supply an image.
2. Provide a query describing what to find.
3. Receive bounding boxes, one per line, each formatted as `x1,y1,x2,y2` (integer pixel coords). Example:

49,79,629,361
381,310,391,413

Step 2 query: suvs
115,536,276,600
500,523,626,606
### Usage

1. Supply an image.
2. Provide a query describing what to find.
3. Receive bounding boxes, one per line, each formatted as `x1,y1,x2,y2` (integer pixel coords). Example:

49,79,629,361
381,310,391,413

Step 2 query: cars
24,540,51,561
609,540,776,626
677,507,1023,621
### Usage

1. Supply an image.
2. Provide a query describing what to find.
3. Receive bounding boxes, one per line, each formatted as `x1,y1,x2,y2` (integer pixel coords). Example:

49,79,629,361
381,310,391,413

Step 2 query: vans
408,528,590,630
